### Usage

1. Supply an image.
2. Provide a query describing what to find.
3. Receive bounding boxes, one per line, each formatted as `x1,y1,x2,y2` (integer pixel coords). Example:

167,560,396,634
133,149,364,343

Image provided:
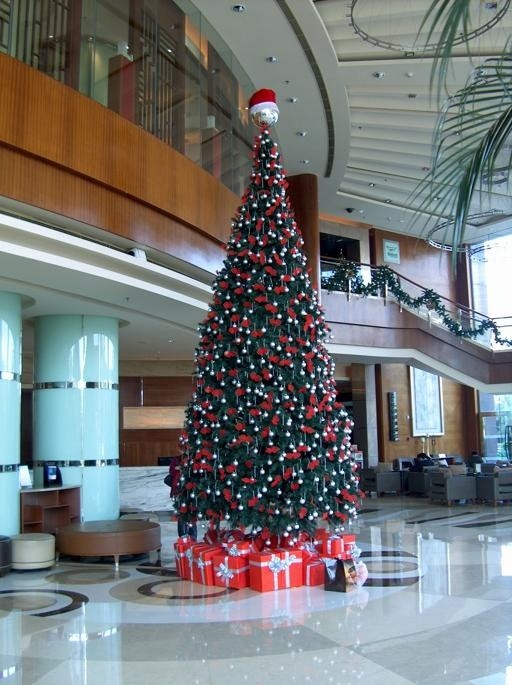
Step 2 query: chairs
360,458,511,507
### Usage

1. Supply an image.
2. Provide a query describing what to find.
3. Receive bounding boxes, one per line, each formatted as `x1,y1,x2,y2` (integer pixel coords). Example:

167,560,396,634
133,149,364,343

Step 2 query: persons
467,450,483,466
409,453,434,472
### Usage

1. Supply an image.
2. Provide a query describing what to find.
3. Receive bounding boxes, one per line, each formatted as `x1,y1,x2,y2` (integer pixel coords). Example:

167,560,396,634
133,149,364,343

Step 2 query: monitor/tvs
43,464,62,488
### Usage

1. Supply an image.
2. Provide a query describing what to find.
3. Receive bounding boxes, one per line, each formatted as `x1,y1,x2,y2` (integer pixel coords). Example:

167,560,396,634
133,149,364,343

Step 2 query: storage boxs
173,528,357,592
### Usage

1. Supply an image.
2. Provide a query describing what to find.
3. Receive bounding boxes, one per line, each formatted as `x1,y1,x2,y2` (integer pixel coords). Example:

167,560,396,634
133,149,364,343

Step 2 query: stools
0,514,160,577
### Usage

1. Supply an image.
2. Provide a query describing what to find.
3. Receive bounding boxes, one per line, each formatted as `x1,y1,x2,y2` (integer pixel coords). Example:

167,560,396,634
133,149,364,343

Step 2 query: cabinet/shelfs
20,485,80,533
